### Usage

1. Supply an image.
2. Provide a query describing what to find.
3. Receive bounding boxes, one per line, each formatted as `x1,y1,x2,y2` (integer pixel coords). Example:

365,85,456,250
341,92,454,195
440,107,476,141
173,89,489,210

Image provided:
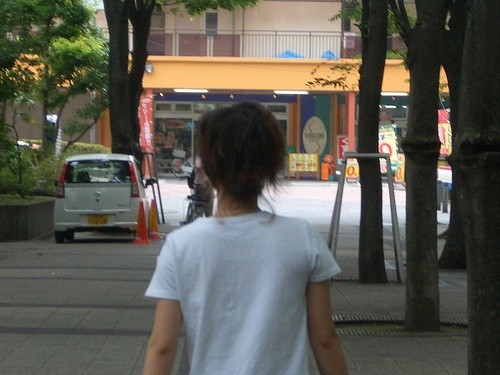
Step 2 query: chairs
77,170,91,183
108,167,127,183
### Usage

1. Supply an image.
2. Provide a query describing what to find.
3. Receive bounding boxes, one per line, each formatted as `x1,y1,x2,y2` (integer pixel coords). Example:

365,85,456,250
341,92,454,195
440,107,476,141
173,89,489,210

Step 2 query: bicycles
179,179,209,228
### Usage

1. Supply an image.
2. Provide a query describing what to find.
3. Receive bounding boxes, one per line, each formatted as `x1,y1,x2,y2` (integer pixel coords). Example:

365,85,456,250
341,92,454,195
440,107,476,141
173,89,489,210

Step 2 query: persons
142,102,351,372
187,155,214,219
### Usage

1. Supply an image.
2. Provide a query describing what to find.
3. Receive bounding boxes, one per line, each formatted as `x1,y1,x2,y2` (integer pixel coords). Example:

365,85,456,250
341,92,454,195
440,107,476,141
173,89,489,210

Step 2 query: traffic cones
128,201,152,245
147,199,161,240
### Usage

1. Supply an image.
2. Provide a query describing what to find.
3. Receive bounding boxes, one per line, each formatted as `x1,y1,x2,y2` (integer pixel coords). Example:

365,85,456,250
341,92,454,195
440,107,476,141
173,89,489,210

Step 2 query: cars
53,153,157,243
